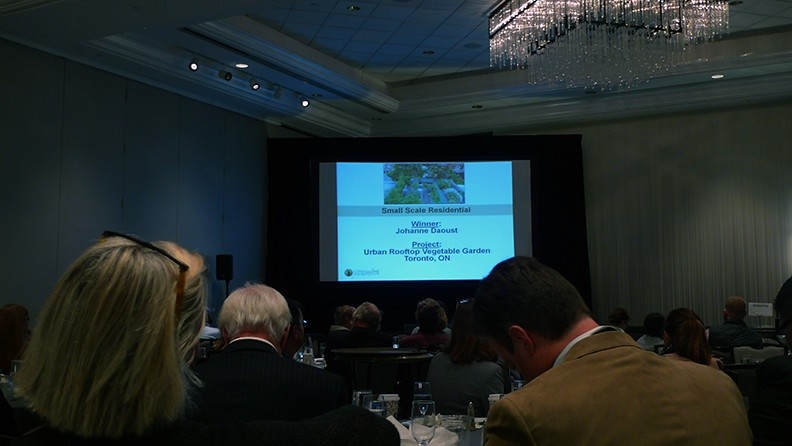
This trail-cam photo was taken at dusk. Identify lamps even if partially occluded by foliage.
[485,1,730,96]
[187,57,310,108]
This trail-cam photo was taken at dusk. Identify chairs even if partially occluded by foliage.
[329,337,792,446]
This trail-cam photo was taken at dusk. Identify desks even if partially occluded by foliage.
[395,413,486,446]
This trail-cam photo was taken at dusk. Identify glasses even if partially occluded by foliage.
[295,320,307,328]
[456,299,471,308]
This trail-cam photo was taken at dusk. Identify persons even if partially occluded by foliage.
[607,307,630,330]
[427,294,510,418]
[471,256,756,446]
[179,282,355,420]
[746,276,792,446]
[0,227,402,446]
[279,298,452,361]
[636,313,666,354]
[0,303,33,375]
[709,295,762,363]
[658,307,725,371]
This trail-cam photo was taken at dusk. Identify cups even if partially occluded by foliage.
[457,417,484,446]
[363,395,386,419]
[488,394,508,408]
[351,390,372,410]
[378,394,400,420]
[293,345,327,370]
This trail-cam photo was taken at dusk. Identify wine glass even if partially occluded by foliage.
[414,381,432,417]
[411,400,436,446]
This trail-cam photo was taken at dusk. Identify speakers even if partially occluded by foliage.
[216,255,234,281]
